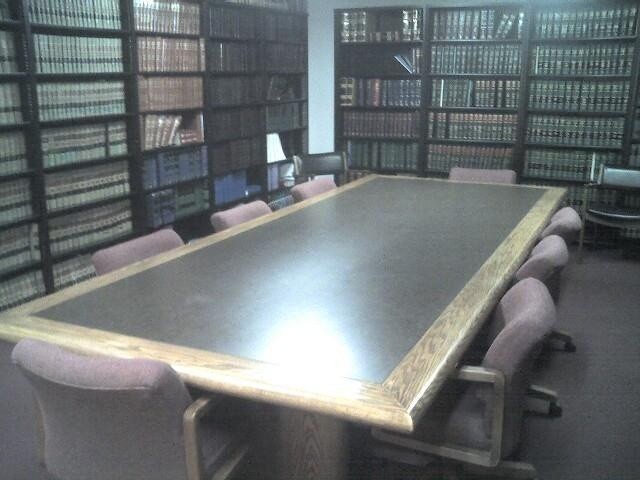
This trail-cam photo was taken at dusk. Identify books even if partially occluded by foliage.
[531,77,638,112]
[426,145,515,172]
[0,121,128,176]
[430,43,523,76]
[208,105,307,141]
[143,149,209,187]
[137,35,206,72]
[141,112,206,146]
[216,155,303,202]
[428,111,517,142]
[565,185,639,240]
[339,8,424,43]
[138,75,205,110]
[206,1,306,43]
[149,182,212,227]
[0,0,125,30]
[0,32,126,76]
[341,110,421,140]
[537,5,640,37]
[527,114,639,145]
[526,148,640,183]
[0,160,132,224]
[1,262,73,311]
[0,198,134,286]
[532,42,633,75]
[214,132,287,170]
[0,79,128,125]
[341,78,422,108]
[343,140,419,170]
[432,78,523,109]
[211,40,304,73]
[129,0,202,34]
[213,74,303,105]
[394,47,422,75]
[432,7,525,39]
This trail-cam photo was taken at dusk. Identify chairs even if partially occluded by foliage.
[363,277,558,480]
[514,235,570,282]
[540,207,581,238]
[292,151,349,192]
[12,338,264,480]
[580,165,640,249]
[92,226,185,274]
[211,199,273,231]
[290,177,339,202]
[446,167,516,187]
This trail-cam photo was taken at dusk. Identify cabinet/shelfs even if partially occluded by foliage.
[0,0,308,310]
[334,1,640,239]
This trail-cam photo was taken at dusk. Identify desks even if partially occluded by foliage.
[0,174,569,480]
